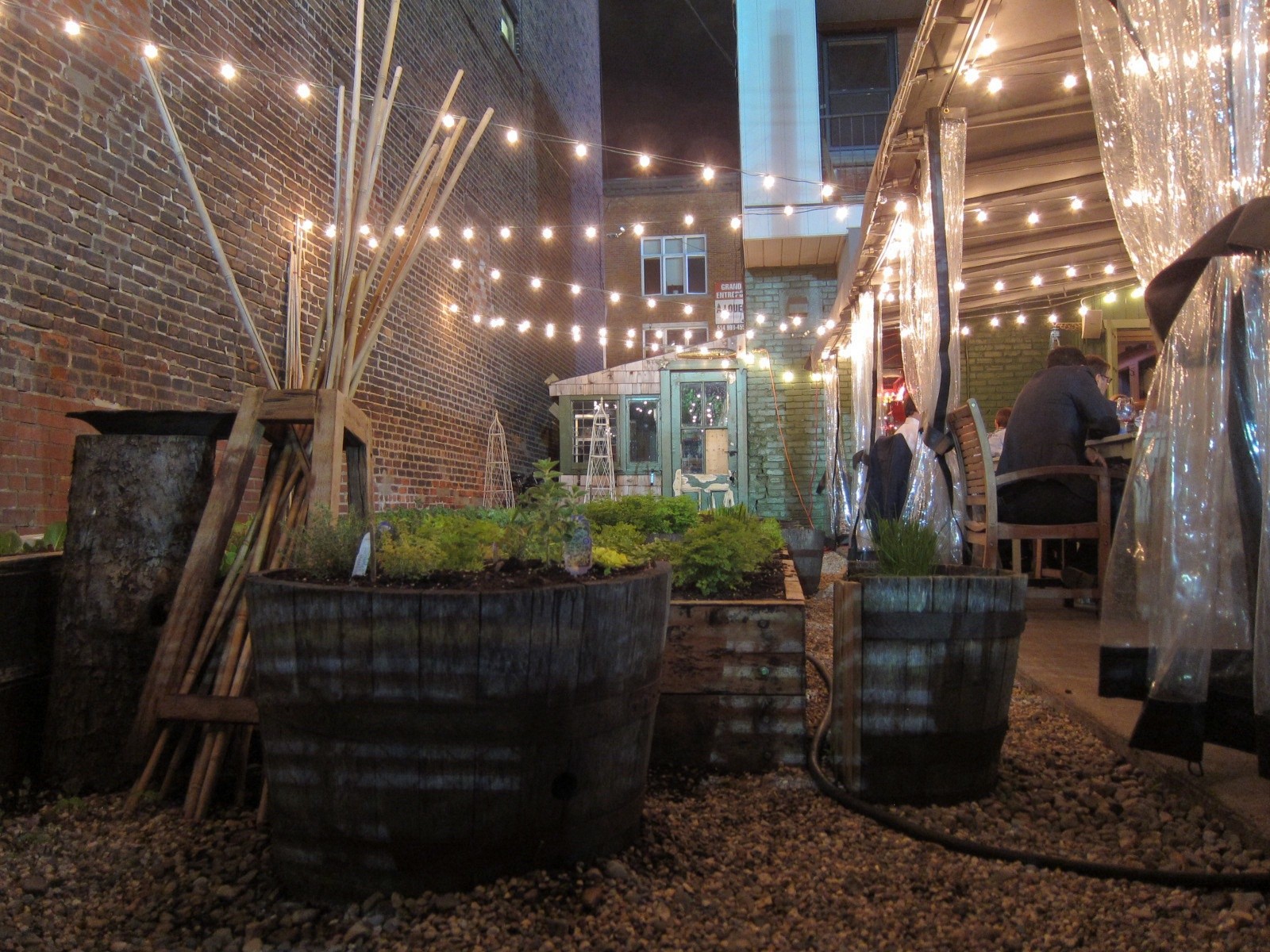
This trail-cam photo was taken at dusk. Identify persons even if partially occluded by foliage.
[989,346,1129,588]
[893,396,919,454]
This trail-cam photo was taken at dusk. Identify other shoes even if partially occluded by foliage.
[1061,567,1096,588]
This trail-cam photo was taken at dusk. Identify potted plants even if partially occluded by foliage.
[240,453,807,902]
[829,502,1028,808]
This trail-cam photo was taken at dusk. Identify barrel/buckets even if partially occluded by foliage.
[782,528,824,597]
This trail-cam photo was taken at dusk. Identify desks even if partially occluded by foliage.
[1084,431,1137,460]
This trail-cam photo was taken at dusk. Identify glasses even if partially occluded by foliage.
[1102,375,1112,385]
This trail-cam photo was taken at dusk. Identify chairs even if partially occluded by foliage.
[945,394,1131,618]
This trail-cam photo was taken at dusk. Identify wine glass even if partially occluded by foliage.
[1117,397,1136,434]
